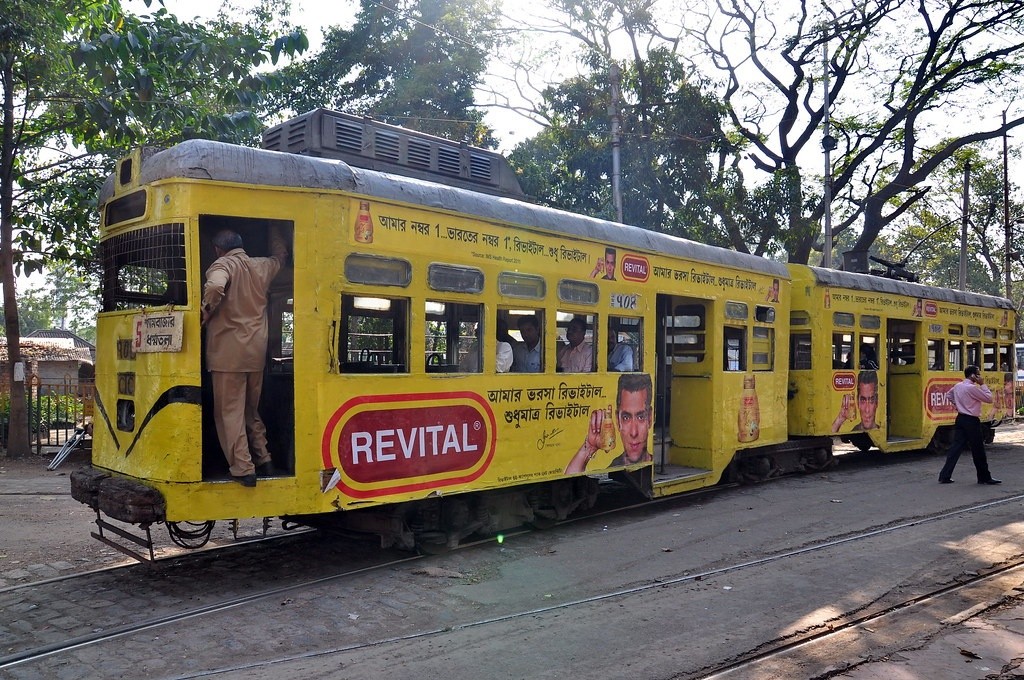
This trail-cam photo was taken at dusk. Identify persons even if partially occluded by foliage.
[556,318,593,373]
[1000,311,1008,327]
[590,248,617,281]
[608,328,634,372]
[912,298,922,317]
[511,315,541,373]
[831,370,879,432]
[988,373,1013,421]
[564,373,653,475]
[496,318,517,349]
[87,418,93,436]
[200,221,288,488]
[462,318,513,373]
[765,279,779,304]
[991,353,1007,371]
[845,343,879,370]
[887,342,915,365]
[938,366,1002,484]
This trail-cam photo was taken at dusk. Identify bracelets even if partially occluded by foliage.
[585,435,596,460]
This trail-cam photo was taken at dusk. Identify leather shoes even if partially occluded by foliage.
[977,477,1002,484]
[939,478,954,483]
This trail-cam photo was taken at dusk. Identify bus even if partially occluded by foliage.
[832,332,1024,381]
[69,109,1015,569]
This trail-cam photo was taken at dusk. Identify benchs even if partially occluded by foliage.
[68,439,93,450]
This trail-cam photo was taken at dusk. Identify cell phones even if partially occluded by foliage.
[971,374,975,378]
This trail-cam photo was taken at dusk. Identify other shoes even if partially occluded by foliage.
[257,462,276,477]
[234,473,256,487]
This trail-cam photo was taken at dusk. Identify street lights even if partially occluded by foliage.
[1003,95,1017,303]
[821,8,863,268]
